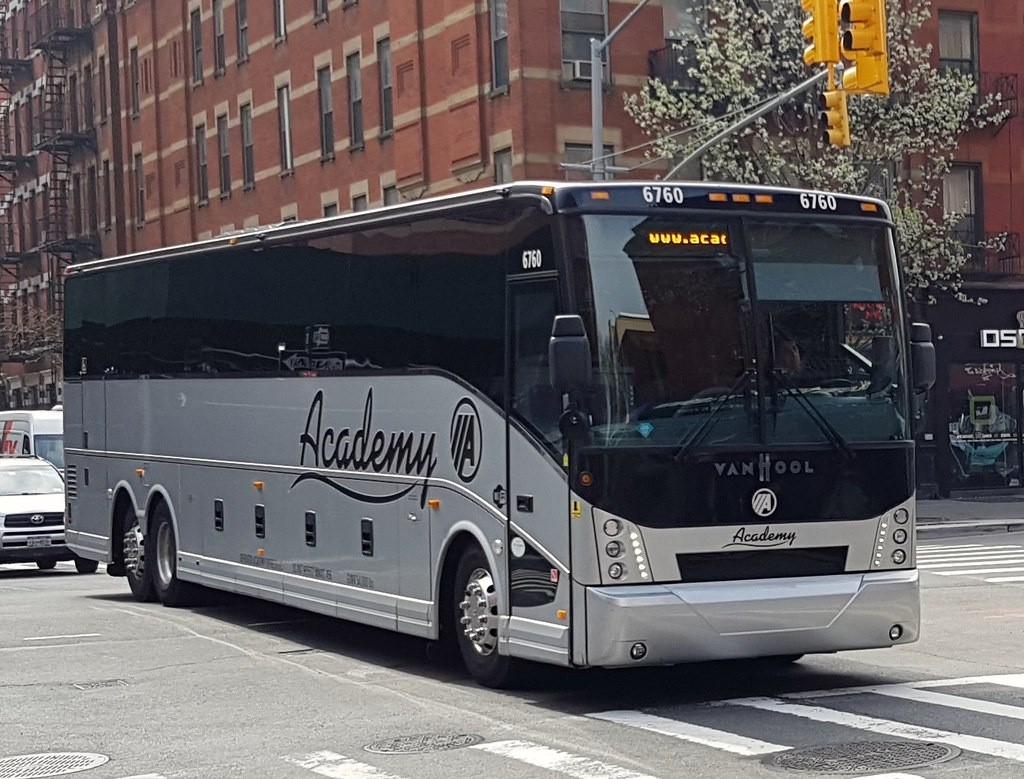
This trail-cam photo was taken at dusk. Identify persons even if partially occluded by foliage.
[37,441,63,465]
[759,331,801,374]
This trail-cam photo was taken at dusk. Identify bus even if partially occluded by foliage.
[62,180,938,689]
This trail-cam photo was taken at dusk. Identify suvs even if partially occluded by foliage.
[0,453,99,575]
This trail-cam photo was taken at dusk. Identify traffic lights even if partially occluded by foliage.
[800,0,840,67]
[819,90,850,150]
[836,0,891,96]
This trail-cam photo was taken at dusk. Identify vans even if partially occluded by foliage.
[0,402,65,481]
